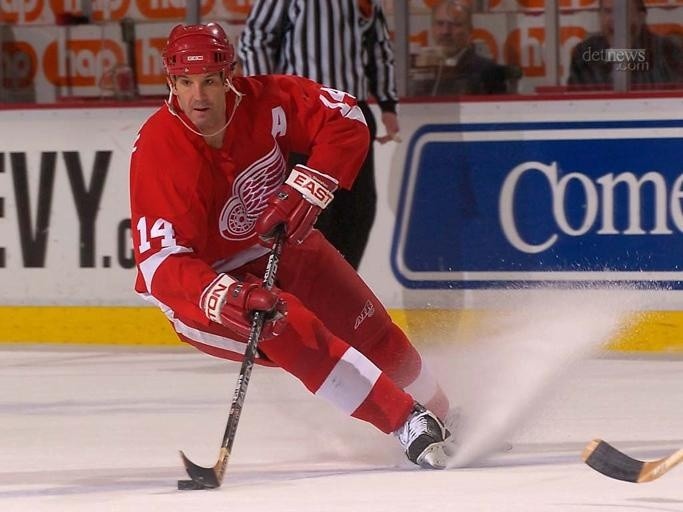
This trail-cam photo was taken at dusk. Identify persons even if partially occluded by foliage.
[407,1,520,99]
[128,22,460,471]
[238,0,401,280]
[563,0,683,87]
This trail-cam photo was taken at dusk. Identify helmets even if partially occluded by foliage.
[162,21,238,90]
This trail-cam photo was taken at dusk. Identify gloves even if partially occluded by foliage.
[198,271,288,341]
[255,163,340,250]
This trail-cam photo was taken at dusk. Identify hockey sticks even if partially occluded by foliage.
[581,439,682,483]
[180,223,285,488]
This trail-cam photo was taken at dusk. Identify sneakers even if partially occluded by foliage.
[396,401,450,466]
